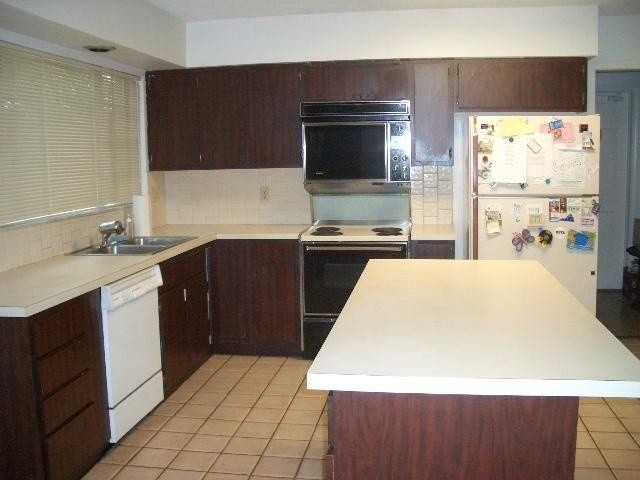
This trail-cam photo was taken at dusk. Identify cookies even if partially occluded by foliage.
[298,100,413,193]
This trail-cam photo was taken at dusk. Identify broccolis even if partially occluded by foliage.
[466,114,602,324]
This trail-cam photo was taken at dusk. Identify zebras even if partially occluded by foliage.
[301,245,409,357]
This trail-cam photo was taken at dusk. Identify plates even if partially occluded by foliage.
[144,54,585,170]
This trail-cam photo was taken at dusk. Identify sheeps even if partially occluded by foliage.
[99,222,124,245]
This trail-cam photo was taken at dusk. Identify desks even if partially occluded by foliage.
[307,256,639,480]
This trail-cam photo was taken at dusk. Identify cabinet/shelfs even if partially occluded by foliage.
[159,241,213,400]
[0,286,111,480]
[208,238,306,357]
[101,264,164,443]
[144,54,585,170]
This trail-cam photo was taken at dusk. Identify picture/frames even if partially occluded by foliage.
[307,256,639,480]
